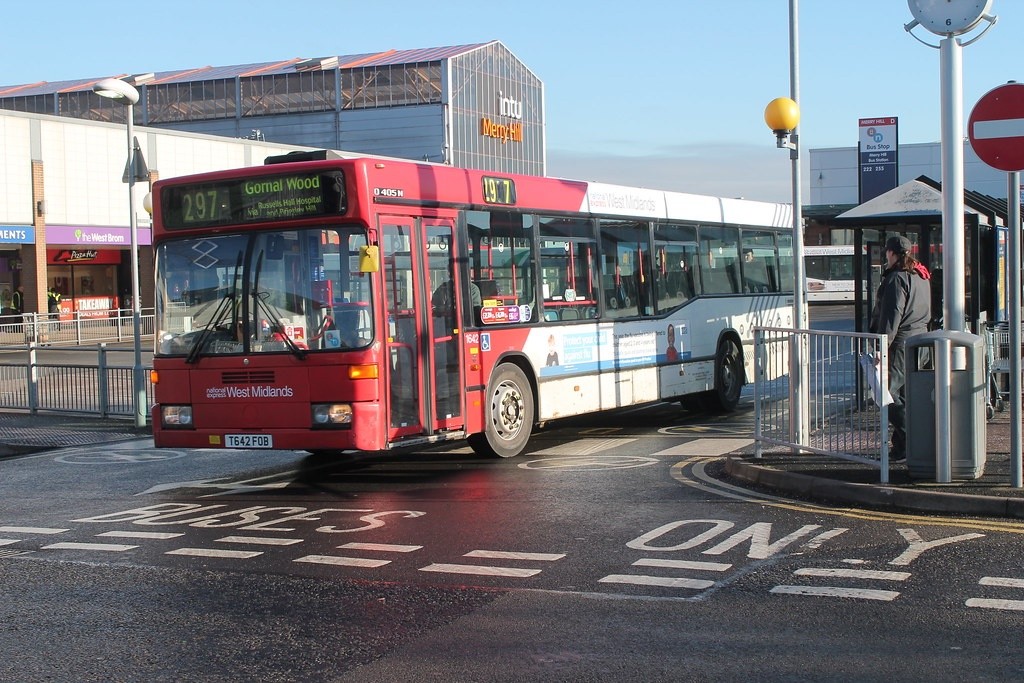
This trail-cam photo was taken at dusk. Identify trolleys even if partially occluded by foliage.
[982,320,1024,419]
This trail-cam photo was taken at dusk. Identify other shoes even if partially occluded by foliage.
[877,454,906,464]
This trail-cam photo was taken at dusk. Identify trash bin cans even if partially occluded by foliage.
[904,330,986,479]
[125,295,142,325]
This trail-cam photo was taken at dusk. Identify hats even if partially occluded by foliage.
[880,237,911,252]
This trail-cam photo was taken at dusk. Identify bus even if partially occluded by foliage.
[148,148,808,463]
[709,248,869,302]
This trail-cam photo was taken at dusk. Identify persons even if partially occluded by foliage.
[667,324,677,362]
[728,247,770,293]
[688,250,729,294]
[545,335,559,367]
[10,286,24,332]
[869,235,931,464]
[48,287,62,331]
[432,257,481,310]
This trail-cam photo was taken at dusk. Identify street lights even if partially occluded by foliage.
[92,79,146,431]
[764,98,808,454]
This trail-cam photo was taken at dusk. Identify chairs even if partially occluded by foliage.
[559,263,778,313]
[470,280,500,326]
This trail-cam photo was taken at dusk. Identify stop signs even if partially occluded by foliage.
[966,83,1024,174]
